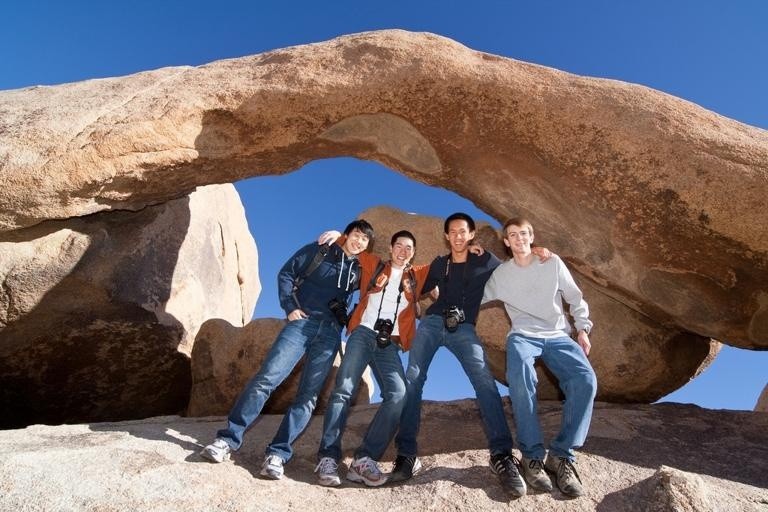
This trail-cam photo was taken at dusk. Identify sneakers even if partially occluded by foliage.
[520,456,553,493]
[261,455,285,480]
[545,454,584,498]
[345,456,387,488]
[201,440,230,463]
[489,454,526,497]
[319,457,343,486]
[389,455,423,481]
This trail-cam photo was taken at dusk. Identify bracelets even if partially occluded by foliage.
[578,326,592,336]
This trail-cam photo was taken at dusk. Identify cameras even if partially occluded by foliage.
[374,319,394,349]
[327,297,349,327]
[442,304,466,333]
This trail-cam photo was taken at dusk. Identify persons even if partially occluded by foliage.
[198,219,375,481]
[427,217,599,497]
[312,230,484,490]
[389,209,553,498]
